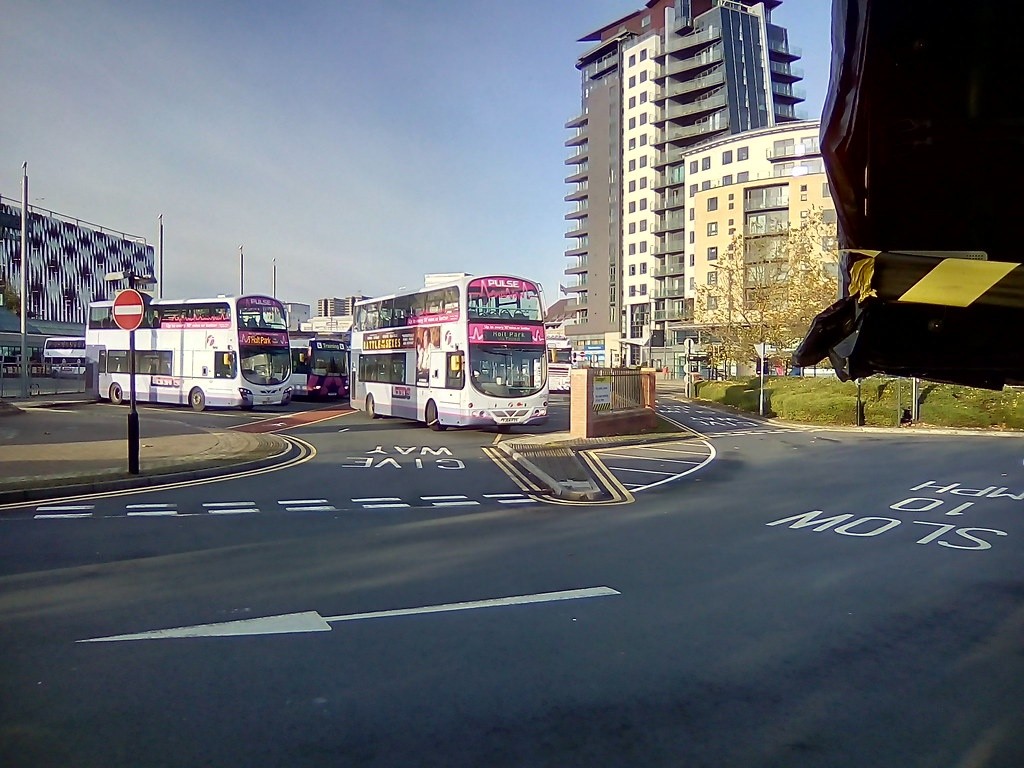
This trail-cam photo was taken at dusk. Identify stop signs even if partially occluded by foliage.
[580,352,585,357]
[112,290,146,334]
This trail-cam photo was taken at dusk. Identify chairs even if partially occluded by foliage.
[259,318,271,328]
[239,318,246,328]
[478,308,525,318]
[152,317,159,327]
[247,318,258,327]
[102,318,150,327]
[362,313,418,330]
[360,364,402,382]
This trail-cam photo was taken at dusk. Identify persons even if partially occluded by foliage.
[662,365,669,381]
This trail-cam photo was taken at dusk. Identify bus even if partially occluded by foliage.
[42,335,86,379]
[82,294,295,413]
[273,337,352,397]
[349,271,558,431]
[546,333,577,392]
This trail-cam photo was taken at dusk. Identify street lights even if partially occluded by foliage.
[708,262,751,380]
